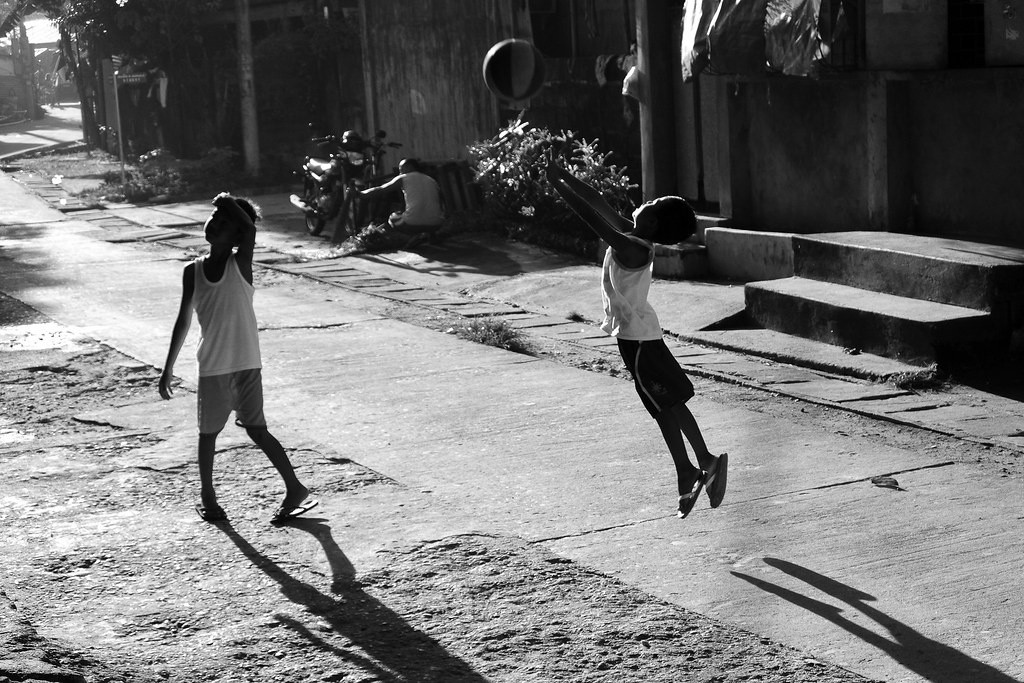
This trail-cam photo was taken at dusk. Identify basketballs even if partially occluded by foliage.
[481,37,550,104]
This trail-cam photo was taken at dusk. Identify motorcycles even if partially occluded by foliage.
[287,122,388,238]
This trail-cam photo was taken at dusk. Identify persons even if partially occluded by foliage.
[358,158,449,238]
[158,192,318,521]
[535,144,728,519]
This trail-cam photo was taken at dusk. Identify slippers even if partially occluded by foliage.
[194,503,228,522]
[269,500,319,523]
[704,453,729,509]
[677,469,708,520]
